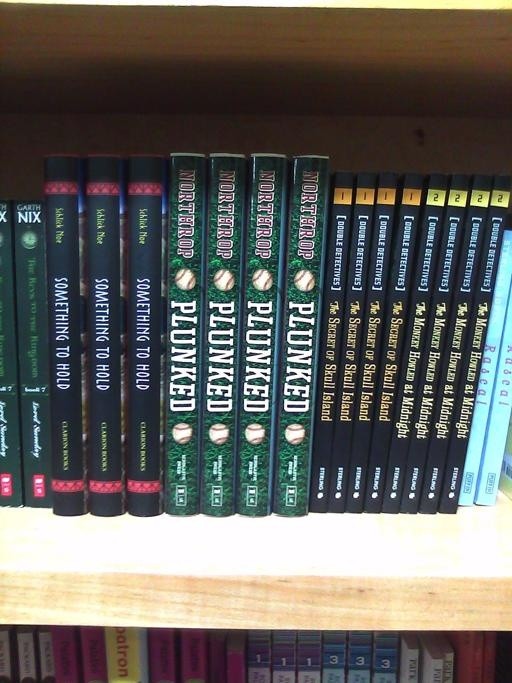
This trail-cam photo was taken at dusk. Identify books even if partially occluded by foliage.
[0,155,512,516]
[0,623,512,683]
